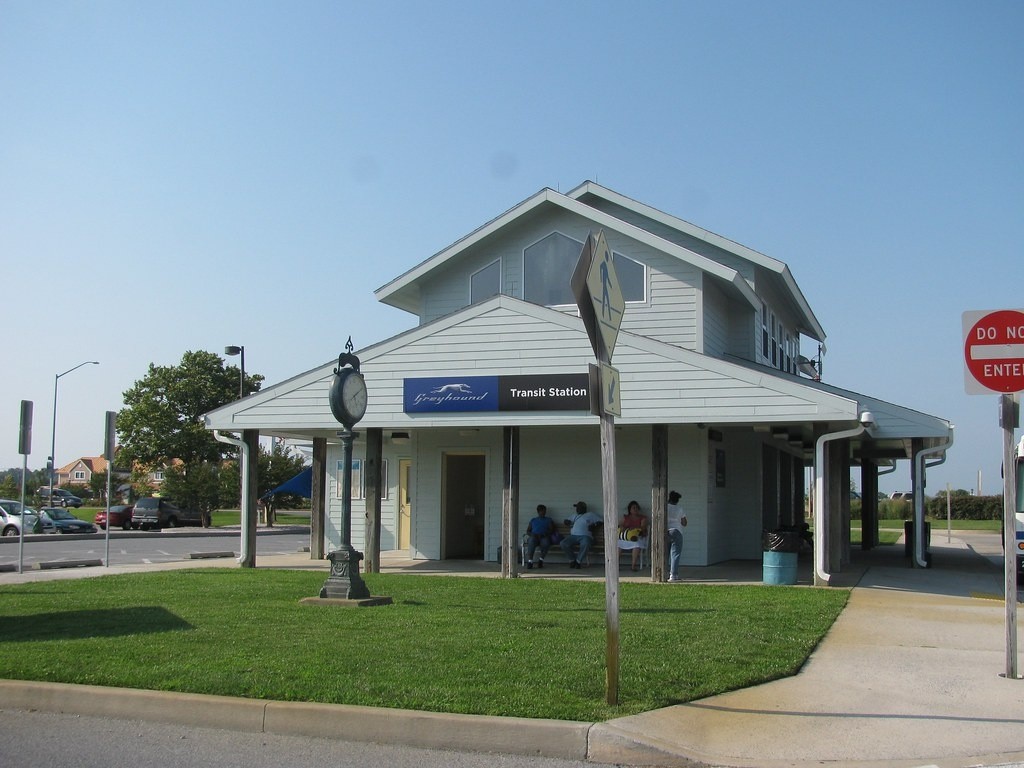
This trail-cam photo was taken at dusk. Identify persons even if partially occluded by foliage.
[560,502,603,570]
[526,505,561,569]
[617,501,648,572]
[666,490,688,583]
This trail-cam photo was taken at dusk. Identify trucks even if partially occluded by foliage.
[1001,434,1024,584]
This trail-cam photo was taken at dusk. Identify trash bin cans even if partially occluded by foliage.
[761,529,801,585]
[904,520,931,568]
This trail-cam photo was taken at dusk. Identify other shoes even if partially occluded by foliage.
[527,562,533,569]
[575,562,581,569]
[570,560,575,567]
[669,575,679,580]
[537,559,543,568]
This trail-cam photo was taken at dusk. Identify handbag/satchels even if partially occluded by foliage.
[551,529,563,544]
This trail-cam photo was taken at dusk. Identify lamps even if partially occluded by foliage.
[786,435,802,447]
[771,428,788,440]
[802,442,814,454]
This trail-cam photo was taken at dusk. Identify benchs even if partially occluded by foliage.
[521,523,650,570]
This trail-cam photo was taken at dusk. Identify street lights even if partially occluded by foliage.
[48,362,100,508]
[225,344,244,400]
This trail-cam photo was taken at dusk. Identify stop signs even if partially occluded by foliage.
[962,310,1024,396]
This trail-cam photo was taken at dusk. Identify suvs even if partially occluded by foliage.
[849,490,913,503]
[34,488,83,509]
[130,497,211,530]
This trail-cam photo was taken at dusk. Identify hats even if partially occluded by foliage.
[669,491,681,498]
[573,502,587,508]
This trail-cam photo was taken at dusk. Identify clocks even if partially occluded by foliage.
[336,371,367,420]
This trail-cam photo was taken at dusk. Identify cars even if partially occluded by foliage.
[95,505,141,530]
[0,499,41,536]
[37,508,98,534]
